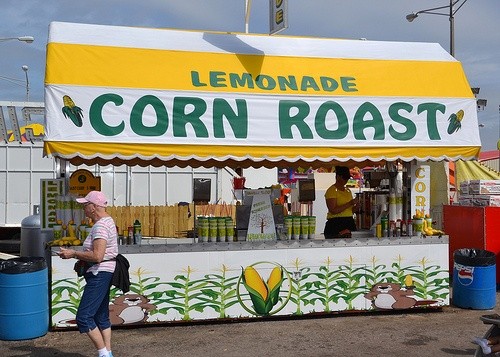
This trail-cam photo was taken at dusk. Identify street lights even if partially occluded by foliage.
[22,65,30,125]
[406,0,467,58]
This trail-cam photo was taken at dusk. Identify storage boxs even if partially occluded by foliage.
[458,180,500,207]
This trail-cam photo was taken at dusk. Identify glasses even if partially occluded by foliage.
[82,201,92,208]
[339,175,350,180]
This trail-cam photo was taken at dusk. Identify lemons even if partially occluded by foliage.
[57,220,95,230]
[53,240,81,246]
[425,227,445,236]
[425,214,430,217]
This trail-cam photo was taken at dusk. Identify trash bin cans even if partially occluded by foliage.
[0,256,49,340]
[452,248,495,310]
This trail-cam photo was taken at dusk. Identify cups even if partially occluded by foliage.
[197,217,233,242]
[120,220,141,246]
[53,224,92,243]
[389,188,403,221]
[283,215,316,240]
[372,217,433,237]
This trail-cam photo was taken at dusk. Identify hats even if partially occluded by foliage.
[75,191,107,208]
[335,166,353,177]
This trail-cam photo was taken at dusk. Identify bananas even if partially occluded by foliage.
[61,237,77,240]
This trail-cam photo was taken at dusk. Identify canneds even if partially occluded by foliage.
[122,226,141,246]
[375,217,401,238]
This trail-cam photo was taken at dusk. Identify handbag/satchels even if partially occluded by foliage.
[73,257,117,277]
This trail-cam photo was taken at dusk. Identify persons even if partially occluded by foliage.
[324,165,361,239]
[59,191,119,357]
[471,335,500,355]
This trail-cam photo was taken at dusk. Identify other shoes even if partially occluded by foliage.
[109,351,113,357]
